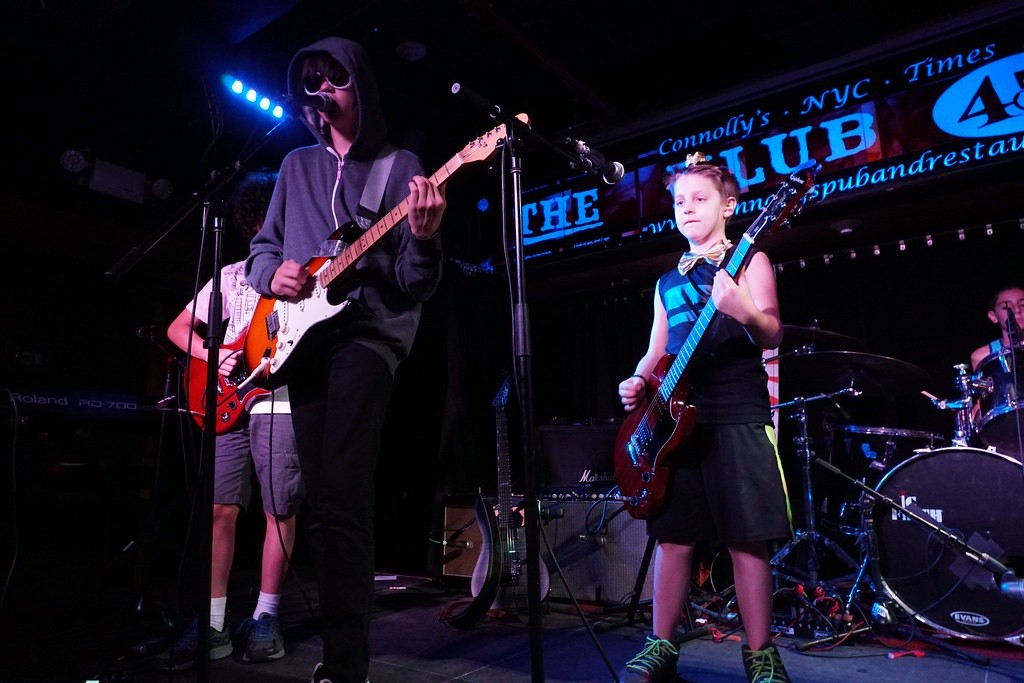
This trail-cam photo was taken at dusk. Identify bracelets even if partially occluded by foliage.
[631,374,647,384]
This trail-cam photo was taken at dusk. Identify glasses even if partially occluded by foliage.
[302,62,352,96]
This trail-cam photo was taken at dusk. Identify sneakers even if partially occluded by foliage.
[237,612,285,660]
[741,642,791,683]
[153,617,234,671]
[311,662,372,683]
[618,632,680,683]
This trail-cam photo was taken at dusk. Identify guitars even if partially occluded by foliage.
[244,112,532,392]
[611,166,814,519]
[471,377,552,610]
[183,321,272,437]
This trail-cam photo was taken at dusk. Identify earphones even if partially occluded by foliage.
[726,205,732,210]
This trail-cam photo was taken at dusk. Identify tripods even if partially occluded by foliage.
[694,365,1007,663]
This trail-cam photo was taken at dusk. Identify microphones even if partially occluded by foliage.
[1006,308,1019,340]
[578,533,607,545]
[443,540,473,549]
[566,137,625,184]
[278,92,332,112]
[1000,568,1024,602]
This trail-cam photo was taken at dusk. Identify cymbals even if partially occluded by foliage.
[782,324,863,345]
[780,351,938,422]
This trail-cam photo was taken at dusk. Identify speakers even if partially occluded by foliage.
[538,492,655,605]
[534,422,625,491]
[443,504,484,578]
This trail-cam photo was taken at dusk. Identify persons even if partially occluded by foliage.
[619,153,793,683]
[970,285,1024,423]
[155,217,307,669]
[244,37,449,683]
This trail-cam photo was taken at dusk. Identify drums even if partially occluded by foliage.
[862,445,1024,641]
[970,341,1024,461]
[819,424,945,537]
